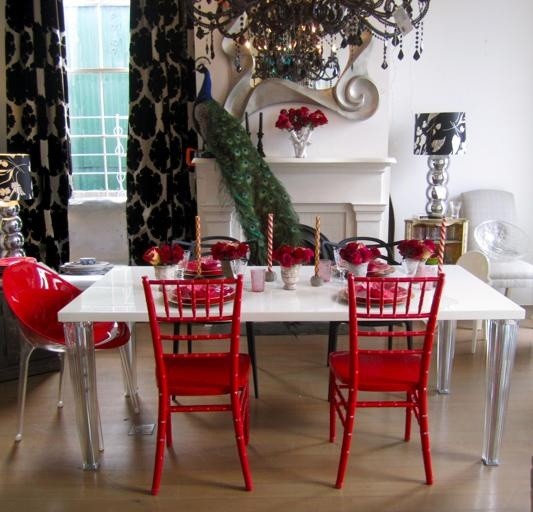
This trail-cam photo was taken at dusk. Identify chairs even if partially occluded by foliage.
[0,260,143,455]
[460,188,533,298]
[436,251,492,353]
[140,275,255,495]
[327,271,447,490]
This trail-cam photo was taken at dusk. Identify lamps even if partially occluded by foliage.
[0,153,35,257]
[413,112,467,216]
[179,1,430,89]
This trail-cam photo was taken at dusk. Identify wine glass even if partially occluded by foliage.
[333,247,346,286]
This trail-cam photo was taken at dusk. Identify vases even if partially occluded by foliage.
[279,264,301,290]
[222,260,249,282]
[404,259,426,277]
[348,262,369,279]
[288,136,312,158]
[154,264,175,291]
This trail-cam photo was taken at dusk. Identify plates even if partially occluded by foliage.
[335,259,414,305]
[167,260,236,305]
[58,260,108,272]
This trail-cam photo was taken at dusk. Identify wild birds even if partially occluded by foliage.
[190,62,303,266]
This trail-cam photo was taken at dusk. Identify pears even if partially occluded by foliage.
[426,257,439,265]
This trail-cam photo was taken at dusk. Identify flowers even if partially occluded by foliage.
[339,241,381,265]
[397,240,436,261]
[143,244,185,266]
[210,242,250,262]
[274,107,328,156]
[271,245,313,267]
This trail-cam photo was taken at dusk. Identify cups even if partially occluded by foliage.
[417,265,439,292]
[400,258,418,277]
[230,259,247,279]
[250,268,266,292]
[178,249,190,270]
[317,258,332,283]
[449,200,462,219]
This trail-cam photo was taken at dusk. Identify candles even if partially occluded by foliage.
[267,213,273,272]
[194,214,201,261]
[437,218,448,274]
[315,215,320,277]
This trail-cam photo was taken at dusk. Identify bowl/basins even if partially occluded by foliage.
[425,259,439,265]
[79,257,95,265]
[474,219,531,259]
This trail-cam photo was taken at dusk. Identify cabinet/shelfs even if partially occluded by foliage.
[0,268,62,382]
[404,217,469,263]
